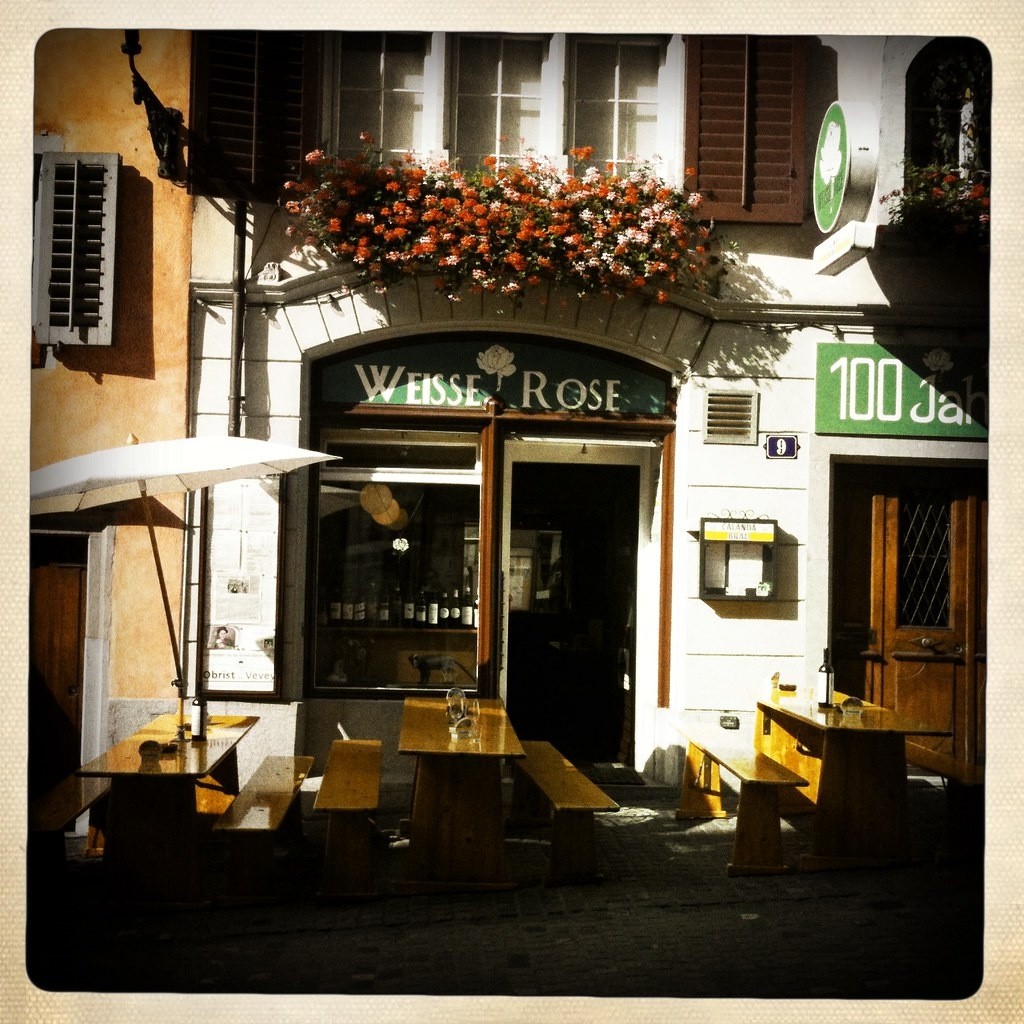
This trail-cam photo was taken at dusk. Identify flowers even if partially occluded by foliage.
[284,133,739,312]
[879,102,991,246]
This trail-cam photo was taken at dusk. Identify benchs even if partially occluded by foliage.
[312,740,384,902]
[27,774,109,912]
[674,727,810,876]
[906,741,984,860]
[210,755,314,908]
[505,740,620,887]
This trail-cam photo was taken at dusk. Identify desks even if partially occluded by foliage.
[72,714,260,912]
[395,696,527,892]
[728,686,952,872]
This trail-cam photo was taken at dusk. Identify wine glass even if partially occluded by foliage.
[446,688,480,743]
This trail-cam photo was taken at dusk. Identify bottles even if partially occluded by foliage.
[426,592,438,628]
[415,591,426,628]
[461,587,473,629]
[403,589,414,628]
[438,592,450,629]
[473,587,479,628]
[317,580,402,627]
[449,589,461,629]
[818,648,834,707]
[191,681,208,742]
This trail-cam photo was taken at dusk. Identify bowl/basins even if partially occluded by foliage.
[160,743,179,753]
[779,684,796,691]
